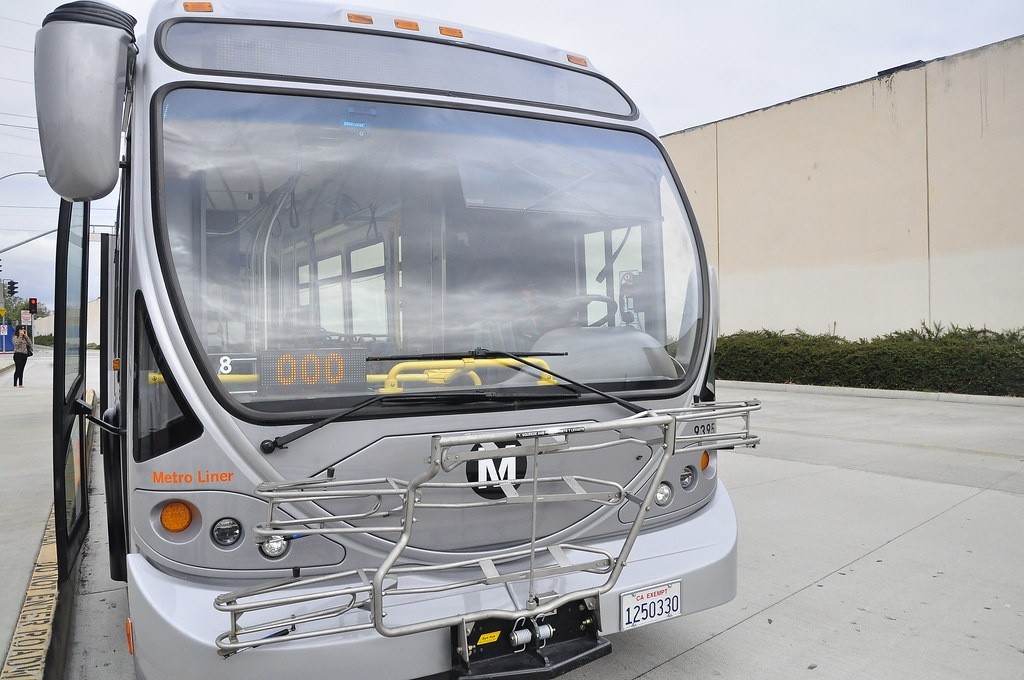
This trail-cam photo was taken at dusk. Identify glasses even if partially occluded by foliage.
[20,328,26,330]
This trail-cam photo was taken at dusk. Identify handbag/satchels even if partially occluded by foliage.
[26,343,33,358]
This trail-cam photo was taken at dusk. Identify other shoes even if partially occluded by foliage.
[14,385,28,389]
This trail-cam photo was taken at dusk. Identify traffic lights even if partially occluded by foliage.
[29,297,37,315]
[0,265,2,267]
[0,270,2,272]
[0,259,1,261]
[12,282,18,296]
[8,280,13,294]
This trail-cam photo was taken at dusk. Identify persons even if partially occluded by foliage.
[12,325,33,388]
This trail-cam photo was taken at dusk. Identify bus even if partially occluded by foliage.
[32,0,762,679]
